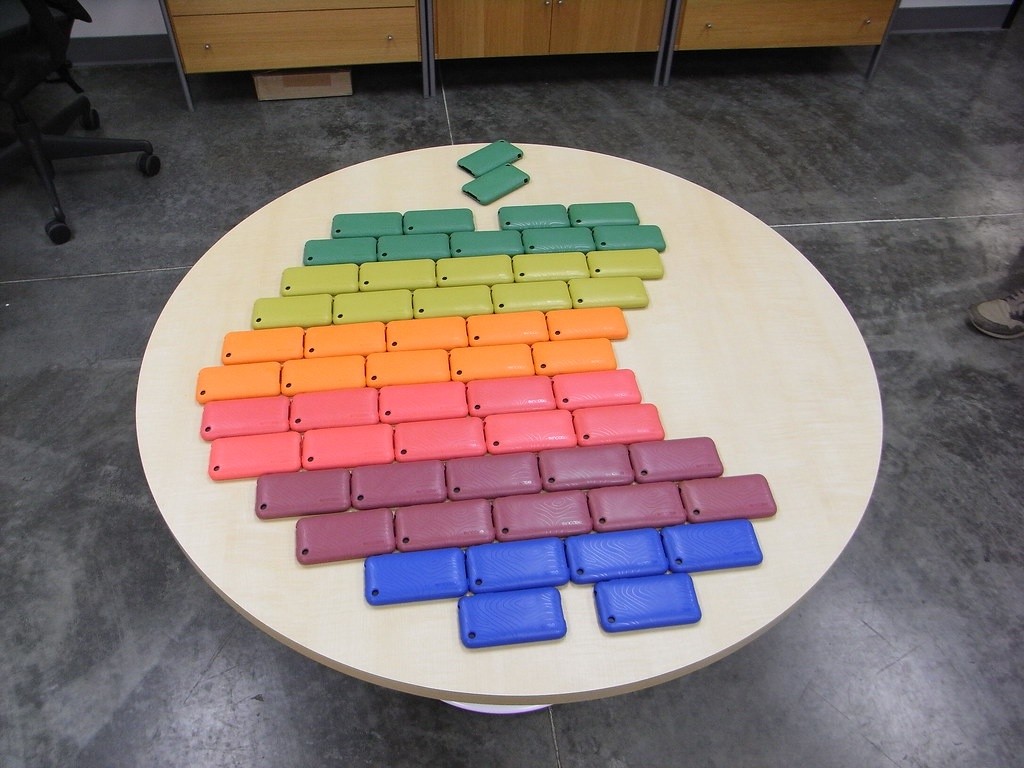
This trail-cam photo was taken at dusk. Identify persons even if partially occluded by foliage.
[969,287,1024,340]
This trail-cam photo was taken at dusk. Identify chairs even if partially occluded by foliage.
[0,0,159,245]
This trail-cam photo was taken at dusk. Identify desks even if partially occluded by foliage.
[135,143,884,713]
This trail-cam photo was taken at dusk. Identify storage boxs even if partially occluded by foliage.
[254,67,354,101]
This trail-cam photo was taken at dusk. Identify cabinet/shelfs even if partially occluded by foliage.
[159,0,901,114]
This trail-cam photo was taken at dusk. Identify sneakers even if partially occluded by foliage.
[967,287,1024,337]
